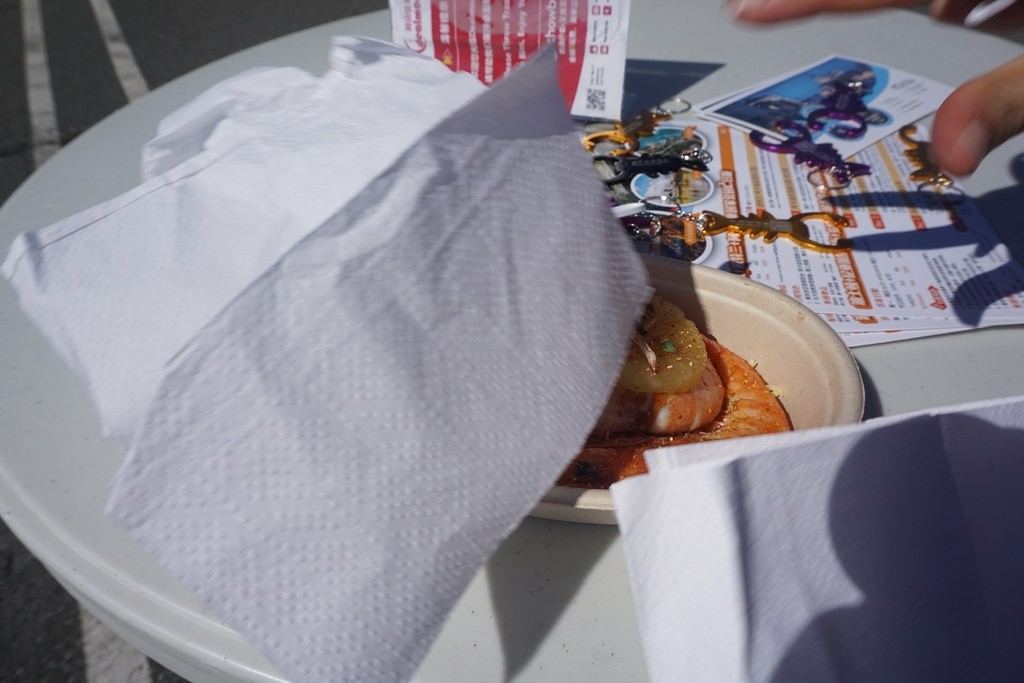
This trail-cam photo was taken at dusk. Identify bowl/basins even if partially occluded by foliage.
[527,250,865,526]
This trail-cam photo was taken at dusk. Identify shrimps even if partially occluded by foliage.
[556,294,801,491]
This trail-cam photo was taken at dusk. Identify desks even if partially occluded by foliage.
[0,1,1024,681]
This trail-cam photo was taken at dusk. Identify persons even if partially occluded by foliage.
[727,0,1024,177]
